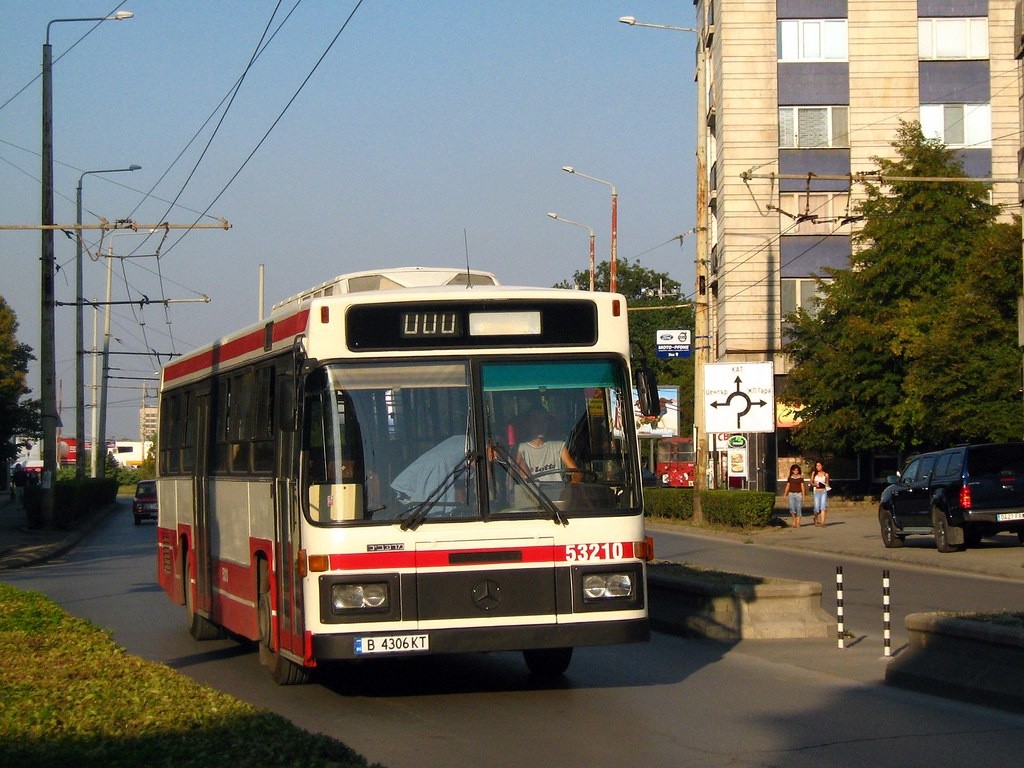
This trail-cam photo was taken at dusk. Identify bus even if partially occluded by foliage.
[656,436,694,489]
[9,459,44,487]
[149,261,664,689]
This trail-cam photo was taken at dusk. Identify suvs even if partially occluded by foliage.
[877,441,1024,553]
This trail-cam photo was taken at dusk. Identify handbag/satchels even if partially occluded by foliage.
[809,471,817,492]
[11,490,16,500]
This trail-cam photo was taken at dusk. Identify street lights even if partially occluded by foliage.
[561,164,619,293]
[74,162,144,521]
[547,211,595,293]
[37,9,138,537]
[94,228,161,475]
[618,15,716,364]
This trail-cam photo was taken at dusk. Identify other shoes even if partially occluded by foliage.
[796,521,800,528]
[792,519,796,528]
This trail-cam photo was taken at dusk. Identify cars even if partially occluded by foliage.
[132,478,158,526]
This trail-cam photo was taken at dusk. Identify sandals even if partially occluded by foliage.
[821,523,826,528]
[814,518,818,528]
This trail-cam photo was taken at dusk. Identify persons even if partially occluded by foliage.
[783,464,806,527]
[810,460,829,527]
[516,404,581,483]
[390,435,474,516]
[29,468,39,486]
[13,464,27,507]
